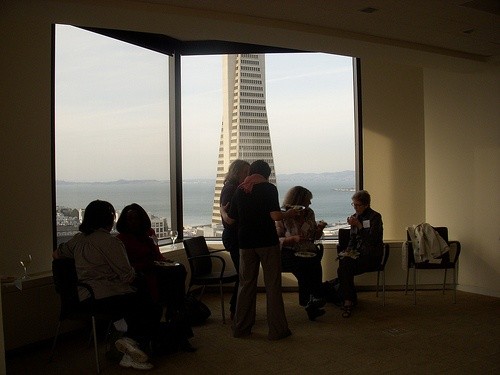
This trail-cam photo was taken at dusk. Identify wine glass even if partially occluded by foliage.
[170,231,178,248]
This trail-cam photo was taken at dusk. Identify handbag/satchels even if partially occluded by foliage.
[322,277,344,305]
[183,293,211,327]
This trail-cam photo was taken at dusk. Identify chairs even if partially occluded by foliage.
[336,228,390,308]
[43,250,119,375]
[182,235,238,324]
[278,238,326,305]
[401,226,461,306]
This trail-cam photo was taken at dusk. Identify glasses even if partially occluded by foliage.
[351,201,363,207]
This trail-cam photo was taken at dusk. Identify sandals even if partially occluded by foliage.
[341,302,353,318]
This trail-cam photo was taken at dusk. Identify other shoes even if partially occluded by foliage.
[120,354,154,370]
[305,305,325,321]
[114,337,148,362]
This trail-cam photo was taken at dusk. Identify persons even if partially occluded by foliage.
[222,160,293,339]
[276,186,323,306]
[337,190,383,317]
[115,204,185,321]
[220,160,251,319]
[53,200,163,370]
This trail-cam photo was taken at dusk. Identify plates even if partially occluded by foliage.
[295,252,316,257]
[281,205,306,211]
